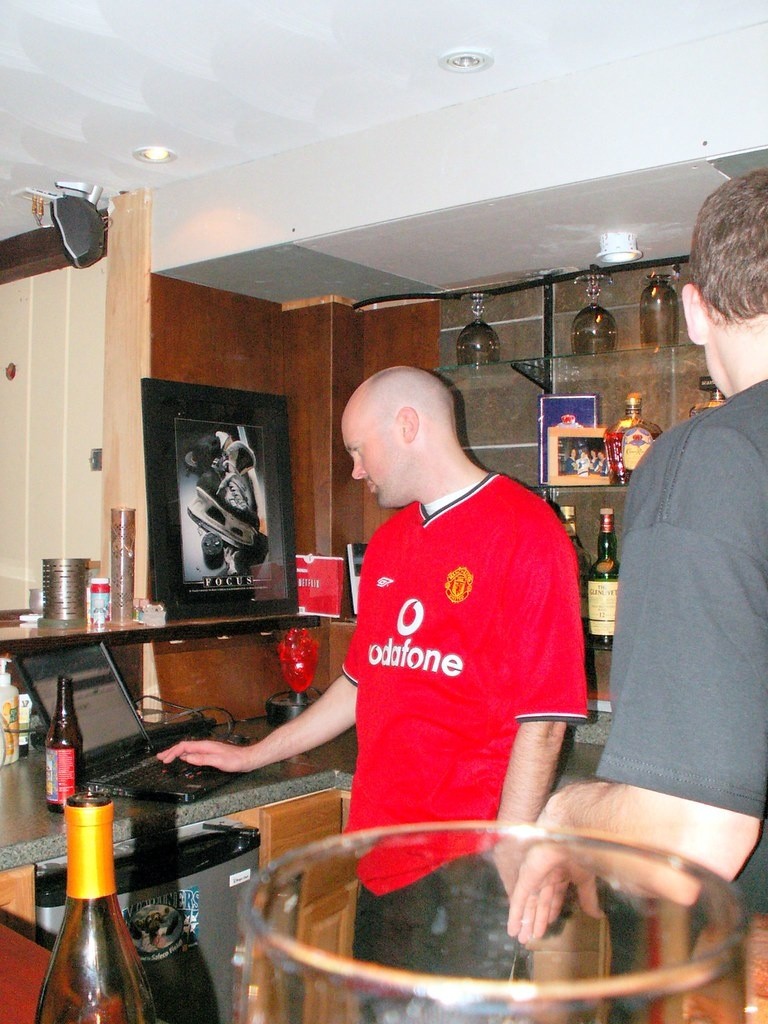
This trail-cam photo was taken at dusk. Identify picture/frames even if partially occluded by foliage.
[141,378,300,621]
[547,427,611,486]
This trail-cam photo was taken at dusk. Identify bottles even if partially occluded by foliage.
[45,676,83,811]
[87,578,110,624]
[602,398,660,484]
[557,506,590,624]
[1,658,20,765]
[640,273,679,347]
[15,682,30,756]
[33,792,158,1024]
[587,507,620,647]
[690,387,728,417]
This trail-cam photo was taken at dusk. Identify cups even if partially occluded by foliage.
[232,820,748,1024]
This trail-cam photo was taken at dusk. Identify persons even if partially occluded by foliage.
[153,366,593,1024]
[506,167,768,947]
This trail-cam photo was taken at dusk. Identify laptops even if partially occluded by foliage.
[11,639,238,802]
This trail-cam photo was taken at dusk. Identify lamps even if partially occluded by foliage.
[49,180,113,270]
[596,231,643,264]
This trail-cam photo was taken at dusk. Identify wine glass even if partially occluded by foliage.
[571,274,617,354]
[456,293,500,366]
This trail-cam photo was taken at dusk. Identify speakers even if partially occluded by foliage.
[49,196,105,269]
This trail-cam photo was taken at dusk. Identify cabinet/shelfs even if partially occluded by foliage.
[0,863,36,943]
[432,341,713,650]
[223,789,359,1024]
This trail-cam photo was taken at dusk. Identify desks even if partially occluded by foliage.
[0,907,129,1024]
